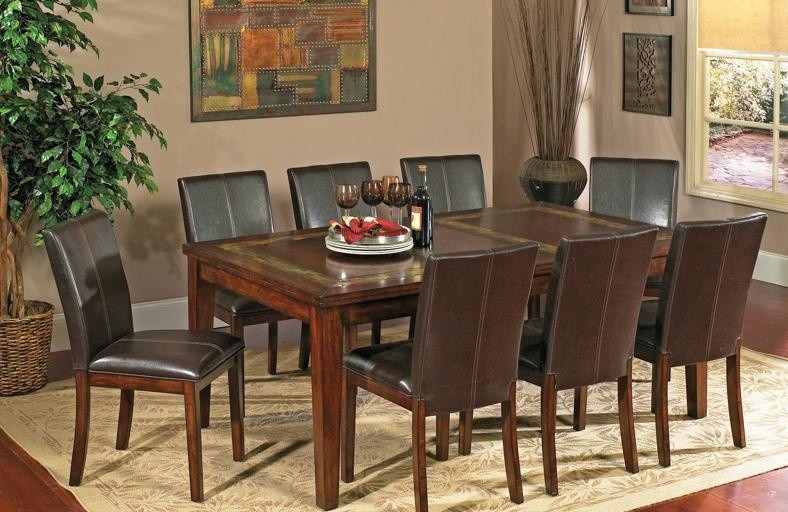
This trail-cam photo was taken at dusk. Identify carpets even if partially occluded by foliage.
[2,322,788,511]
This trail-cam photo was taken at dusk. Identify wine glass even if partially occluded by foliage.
[386,182,410,225]
[334,184,359,215]
[382,176,399,222]
[361,181,383,217]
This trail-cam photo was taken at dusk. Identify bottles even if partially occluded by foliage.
[410,164,433,247]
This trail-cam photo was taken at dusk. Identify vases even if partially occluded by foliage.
[518,156,587,209]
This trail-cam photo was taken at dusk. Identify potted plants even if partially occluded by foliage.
[0,1,167,402]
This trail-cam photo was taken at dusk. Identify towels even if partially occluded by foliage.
[328,214,408,243]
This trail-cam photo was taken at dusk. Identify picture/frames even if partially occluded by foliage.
[186,0,377,122]
[620,31,673,118]
[623,0,672,16]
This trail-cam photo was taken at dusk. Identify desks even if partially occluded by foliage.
[177,195,712,509]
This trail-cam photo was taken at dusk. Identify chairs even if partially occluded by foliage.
[337,242,538,512]
[176,169,297,374]
[39,209,249,503]
[286,161,382,365]
[459,226,657,497]
[397,153,488,217]
[574,211,769,470]
[589,156,682,231]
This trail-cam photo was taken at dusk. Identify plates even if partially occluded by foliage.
[322,236,414,256]
